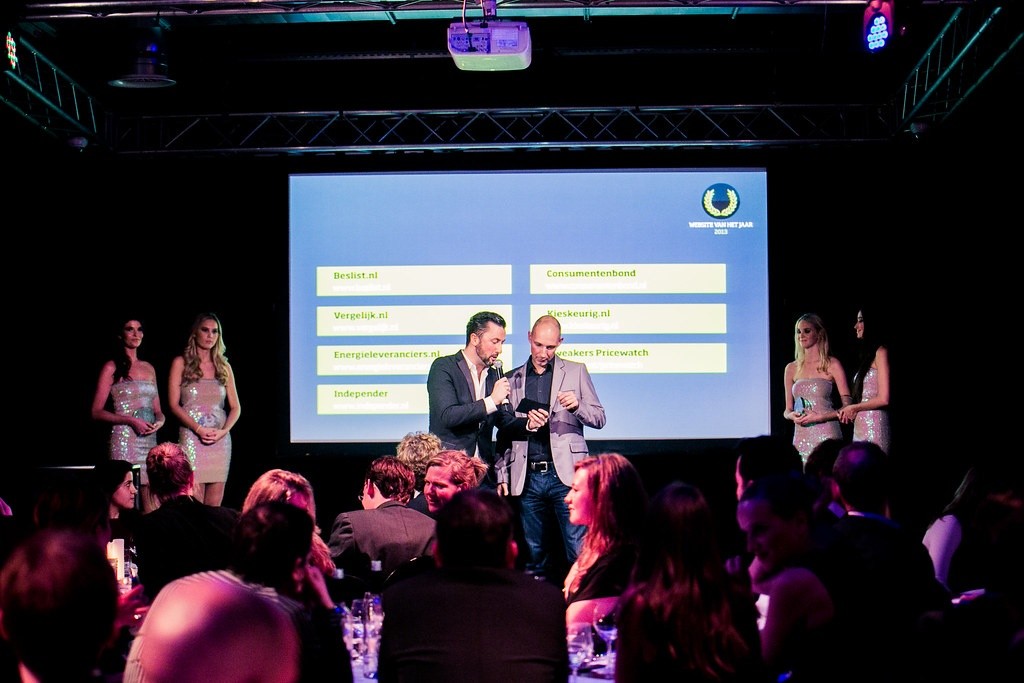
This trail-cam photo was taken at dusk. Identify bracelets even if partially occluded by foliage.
[196,424,201,433]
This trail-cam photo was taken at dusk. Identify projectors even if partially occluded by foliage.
[447,20,531,71]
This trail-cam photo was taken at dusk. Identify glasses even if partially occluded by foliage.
[358,483,367,501]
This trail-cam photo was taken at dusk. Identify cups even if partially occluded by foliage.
[336,593,385,679]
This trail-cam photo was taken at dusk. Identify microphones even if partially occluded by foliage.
[493,360,509,405]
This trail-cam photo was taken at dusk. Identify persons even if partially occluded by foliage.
[0,434,1024,683]
[167,312,243,507]
[426,310,551,489]
[90,313,166,487]
[783,307,891,475]
[493,314,607,578]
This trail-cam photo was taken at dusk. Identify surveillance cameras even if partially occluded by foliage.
[910,123,928,141]
[68,137,87,153]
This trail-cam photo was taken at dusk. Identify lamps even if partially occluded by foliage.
[107,11,176,89]
[863,0,894,53]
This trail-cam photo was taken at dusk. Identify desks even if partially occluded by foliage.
[40,464,140,510]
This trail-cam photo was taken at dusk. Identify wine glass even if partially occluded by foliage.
[565,601,618,682]
